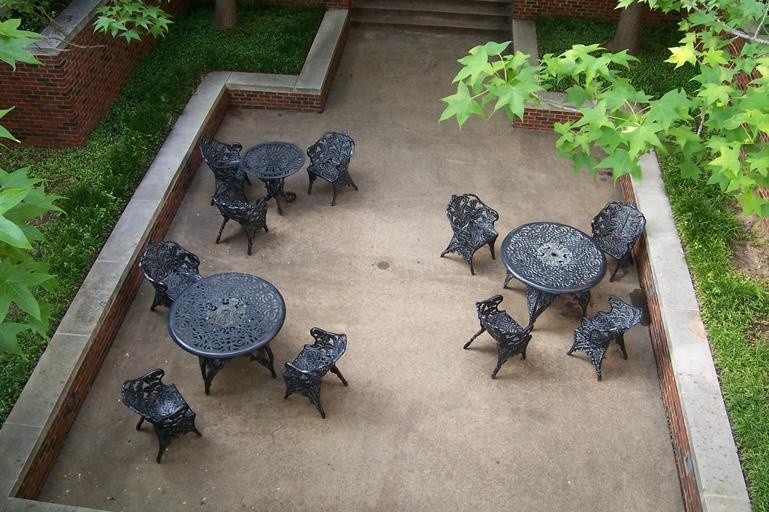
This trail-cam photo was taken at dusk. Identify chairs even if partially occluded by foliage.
[300,129,358,207]
[211,186,268,255]
[283,327,347,417]
[138,239,200,311]
[199,138,252,205]
[464,294,534,380]
[441,193,499,277]
[566,295,643,383]
[591,202,646,283]
[121,368,202,463]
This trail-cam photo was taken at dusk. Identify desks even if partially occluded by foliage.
[167,272,286,396]
[501,222,607,329]
[241,142,305,217]
